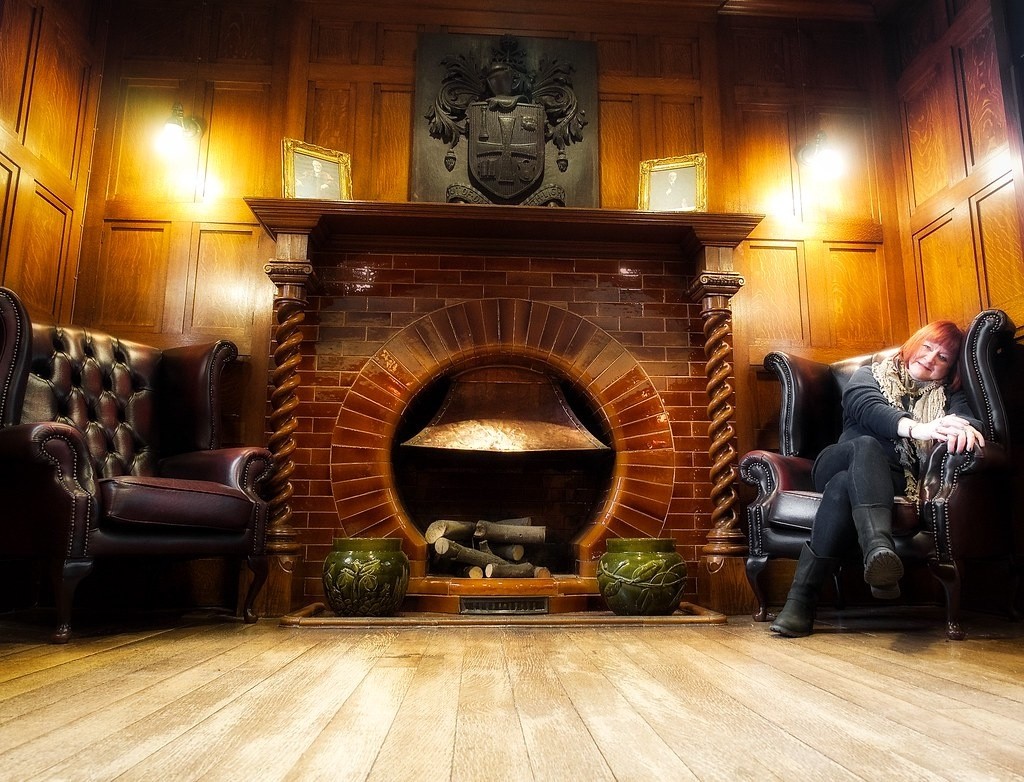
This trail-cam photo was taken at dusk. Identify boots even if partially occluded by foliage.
[770,540,837,638]
[854,503,905,601]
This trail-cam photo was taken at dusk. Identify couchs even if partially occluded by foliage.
[0,285,275,644]
[736,310,1023,640]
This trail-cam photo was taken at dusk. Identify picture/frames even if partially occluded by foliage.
[637,151,706,213]
[279,135,354,201]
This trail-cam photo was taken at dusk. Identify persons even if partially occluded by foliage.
[297,159,337,199]
[655,171,691,212]
[767,317,986,639]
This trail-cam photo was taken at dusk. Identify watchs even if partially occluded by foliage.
[908,420,920,441]
